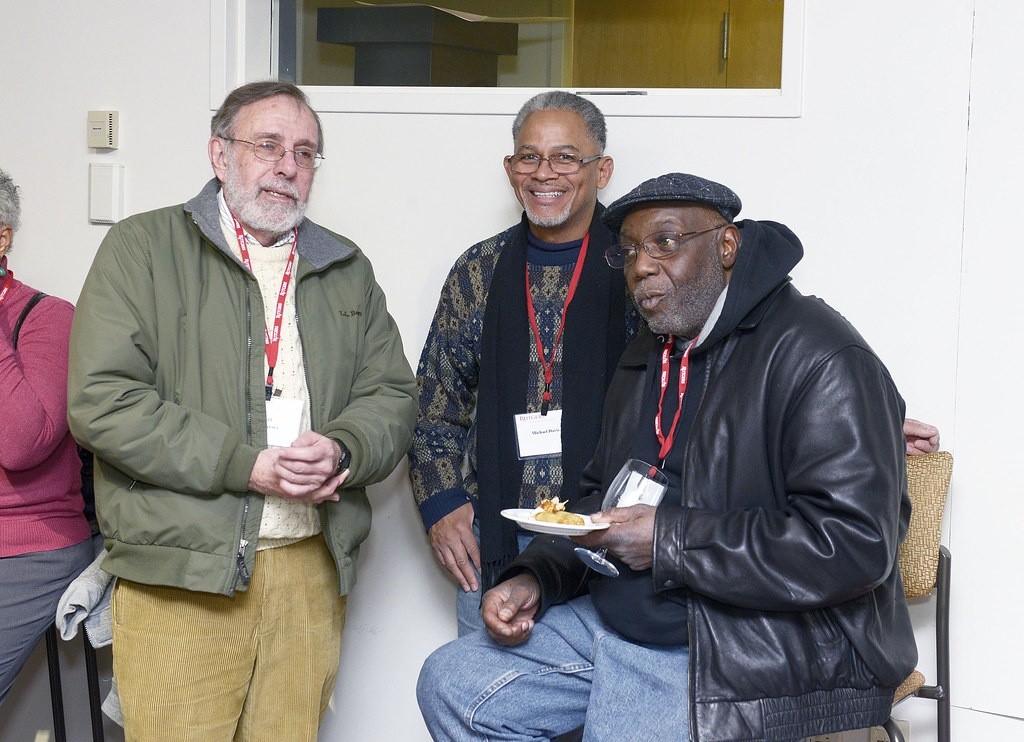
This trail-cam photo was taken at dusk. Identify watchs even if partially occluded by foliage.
[330,437,351,476]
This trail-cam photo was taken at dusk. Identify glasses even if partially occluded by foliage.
[511,152,601,174]
[603,224,727,259]
[222,135,326,170]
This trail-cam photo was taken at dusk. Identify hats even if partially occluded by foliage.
[601,173,743,222]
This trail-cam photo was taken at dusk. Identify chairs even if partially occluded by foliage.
[894,450,951,742]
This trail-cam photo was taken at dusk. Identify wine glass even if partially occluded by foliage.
[574,459,669,576]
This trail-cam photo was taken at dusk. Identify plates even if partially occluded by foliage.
[500,509,611,535]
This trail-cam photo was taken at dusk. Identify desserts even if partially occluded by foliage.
[531,496,585,526]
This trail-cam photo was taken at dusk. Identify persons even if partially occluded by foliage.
[66,83,416,742]
[404,92,940,637]
[0,167,90,703]
[417,173,919,742]
